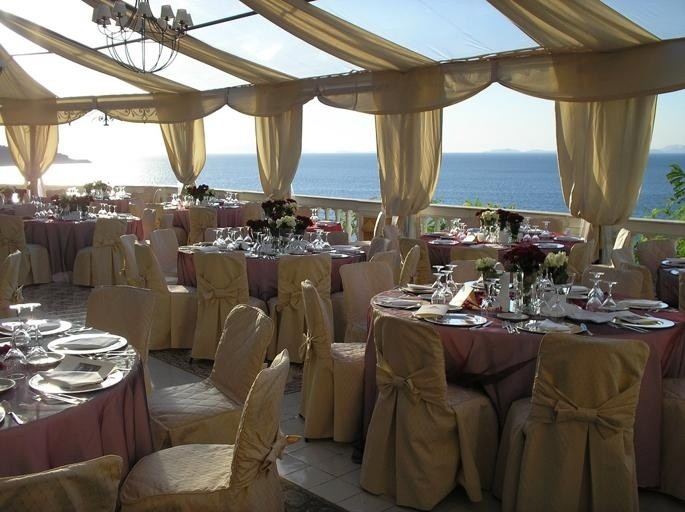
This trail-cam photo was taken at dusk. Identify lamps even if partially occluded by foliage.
[92,0,194,74]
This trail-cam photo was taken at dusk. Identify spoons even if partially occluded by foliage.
[581,322,594,336]
[31,393,80,404]
[3,400,23,425]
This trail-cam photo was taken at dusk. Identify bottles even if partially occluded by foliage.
[514,256,525,289]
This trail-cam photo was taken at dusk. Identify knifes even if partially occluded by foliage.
[481,321,493,328]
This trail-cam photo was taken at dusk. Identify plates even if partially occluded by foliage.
[424,313,487,326]
[375,299,420,308]
[624,316,675,328]
[29,368,123,393]
[569,284,590,300]
[554,235,584,242]
[0,407,5,422]
[618,298,669,309]
[534,242,564,249]
[0,378,16,392]
[470,280,514,291]
[27,352,65,366]
[516,321,583,333]
[47,334,127,355]
[0,319,72,335]
[497,312,528,320]
[429,238,459,245]
[568,311,597,319]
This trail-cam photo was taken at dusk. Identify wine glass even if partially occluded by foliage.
[430,264,458,304]
[33,201,118,218]
[533,266,571,320]
[212,226,334,255]
[64,186,127,199]
[585,270,621,310]
[170,192,238,207]
[3,302,48,381]
[309,208,319,224]
[448,217,551,242]
[1,187,30,204]
